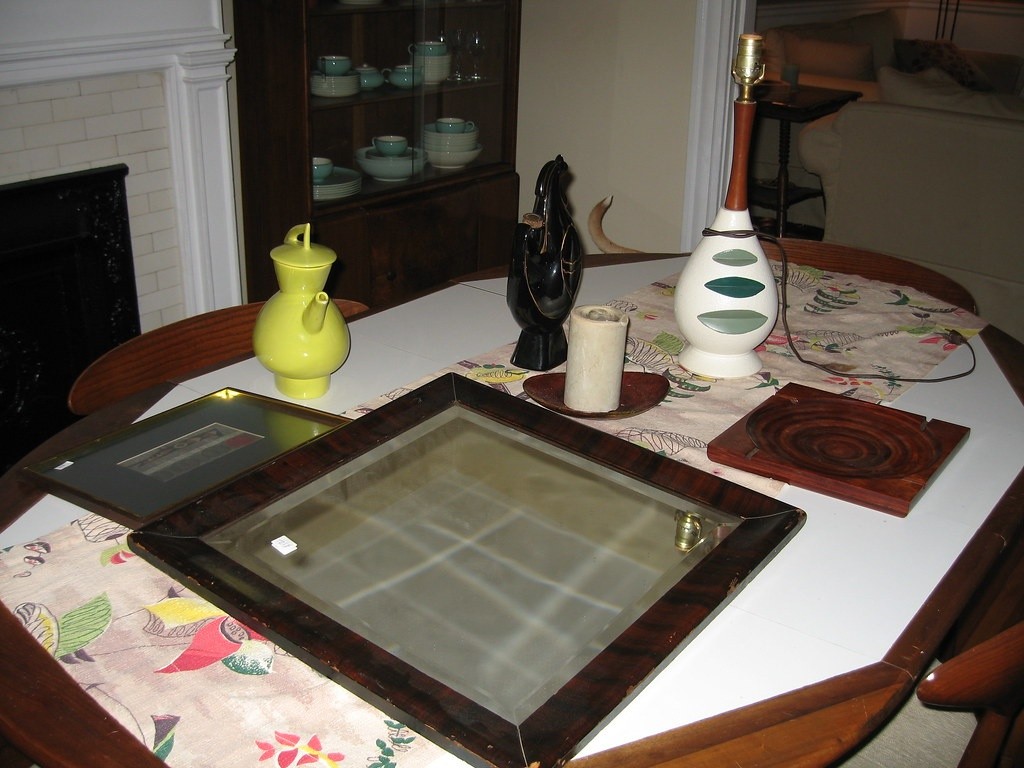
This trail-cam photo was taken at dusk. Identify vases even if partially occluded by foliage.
[674,207,780,379]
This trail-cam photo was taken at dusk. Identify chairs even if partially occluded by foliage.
[826,622,1024,768]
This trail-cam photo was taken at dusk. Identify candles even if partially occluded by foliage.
[563,305,629,414]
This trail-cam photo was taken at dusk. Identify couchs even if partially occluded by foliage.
[752,8,1024,344]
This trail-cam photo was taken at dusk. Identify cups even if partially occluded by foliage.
[781,63,801,86]
[436,117,474,133]
[372,136,408,157]
[564,305,629,412]
[316,55,351,76]
[409,41,448,55]
[313,157,333,182]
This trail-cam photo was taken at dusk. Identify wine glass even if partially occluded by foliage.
[435,29,489,80]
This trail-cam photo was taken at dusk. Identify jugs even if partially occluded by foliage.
[252,224,350,398]
[381,65,425,89]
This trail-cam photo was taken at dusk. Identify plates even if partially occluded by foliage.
[310,71,361,97]
[313,167,361,201]
[420,122,479,151]
[365,148,417,160]
[412,51,452,84]
[523,371,669,420]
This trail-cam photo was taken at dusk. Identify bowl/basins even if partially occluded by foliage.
[356,146,427,182]
[415,142,483,169]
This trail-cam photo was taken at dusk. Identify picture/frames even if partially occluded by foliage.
[17,386,353,530]
[126,372,807,768]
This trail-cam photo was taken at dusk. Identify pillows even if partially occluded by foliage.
[893,36,990,91]
[875,65,1024,120]
[783,33,874,83]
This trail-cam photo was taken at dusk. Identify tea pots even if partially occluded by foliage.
[356,64,385,91]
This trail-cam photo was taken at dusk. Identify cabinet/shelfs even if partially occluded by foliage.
[232,0,522,308]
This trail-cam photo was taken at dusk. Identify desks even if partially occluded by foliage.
[0,255,1024,768]
[748,79,863,241]
